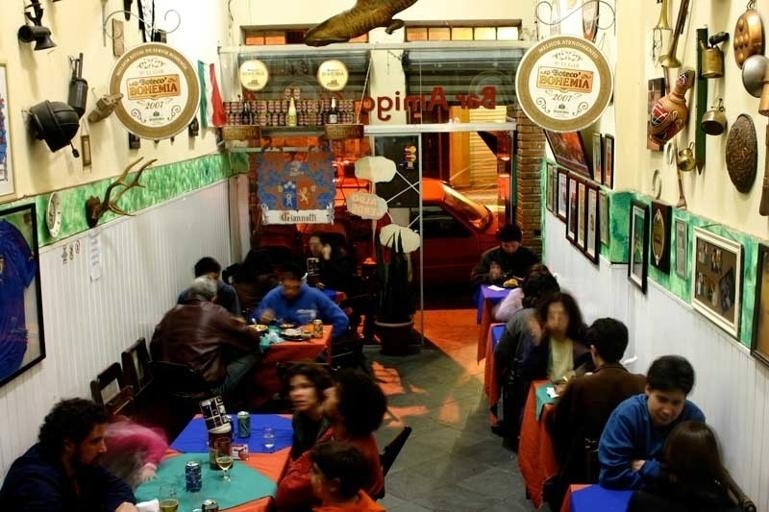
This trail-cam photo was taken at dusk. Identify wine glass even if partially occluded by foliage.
[214,436,235,481]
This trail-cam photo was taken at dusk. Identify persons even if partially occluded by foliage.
[246,270,348,344]
[193,256,240,317]
[308,445,387,512]
[95,419,169,494]
[149,275,263,412]
[505,293,735,512]
[470,226,538,284]
[495,264,560,338]
[274,377,385,510]
[304,232,347,287]
[1,399,138,512]
[278,361,334,459]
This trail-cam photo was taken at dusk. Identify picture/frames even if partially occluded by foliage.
[0,203,48,388]
[750,242,769,369]
[0,62,13,196]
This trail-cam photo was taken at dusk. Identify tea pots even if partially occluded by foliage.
[699,39,723,78]
[700,97,728,136]
[676,141,695,172]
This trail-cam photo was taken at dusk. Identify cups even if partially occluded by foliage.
[261,424,276,449]
[159,483,179,511]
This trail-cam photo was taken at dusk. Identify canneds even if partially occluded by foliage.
[200,500,219,512]
[312,319,324,338]
[184,461,203,492]
[237,411,251,438]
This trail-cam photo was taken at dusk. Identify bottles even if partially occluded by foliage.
[78,117,94,171]
[287,97,297,126]
[328,98,338,125]
[242,102,250,124]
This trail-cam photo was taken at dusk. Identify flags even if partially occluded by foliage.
[197,60,228,129]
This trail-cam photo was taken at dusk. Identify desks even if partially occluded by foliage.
[477,276,754,512]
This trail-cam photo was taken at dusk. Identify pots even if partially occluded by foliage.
[732,0,764,69]
[741,54,768,97]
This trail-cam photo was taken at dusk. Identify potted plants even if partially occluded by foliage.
[373,232,414,354]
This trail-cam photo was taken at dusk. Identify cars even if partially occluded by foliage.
[249,174,505,290]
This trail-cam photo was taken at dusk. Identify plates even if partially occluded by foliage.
[43,192,64,239]
[280,332,305,341]
[250,324,268,332]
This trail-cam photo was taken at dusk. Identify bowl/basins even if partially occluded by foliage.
[279,322,297,329]
